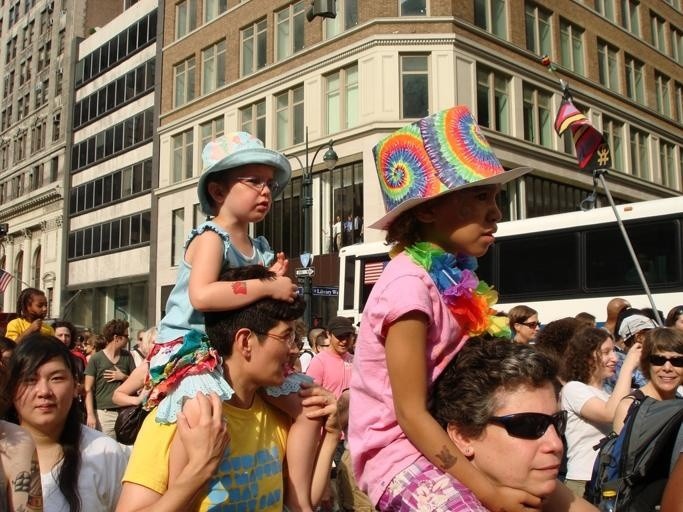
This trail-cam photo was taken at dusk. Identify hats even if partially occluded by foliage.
[367,102,533,231]
[618,314,658,343]
[197,132,292,214]
[327,315,357,338]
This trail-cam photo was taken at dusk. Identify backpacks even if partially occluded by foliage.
[582,394,682,512]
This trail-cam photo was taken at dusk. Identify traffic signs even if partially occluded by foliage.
[293,285,302,296]
[311,287,337,295]
[295,266,315,276]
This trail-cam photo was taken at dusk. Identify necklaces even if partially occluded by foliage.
[347,184,547,512]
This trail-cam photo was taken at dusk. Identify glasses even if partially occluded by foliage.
[485,409,569,439]
[255,330,298,347]
[117,335,129,339]
[520,320,541,329]
[222,174,280,192]
[648,354,682,368]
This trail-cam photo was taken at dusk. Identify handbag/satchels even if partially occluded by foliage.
[114,405,150,445]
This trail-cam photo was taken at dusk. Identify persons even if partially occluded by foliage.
[0,264,682,511]
[333,212,364,253]
[145,130,324,512]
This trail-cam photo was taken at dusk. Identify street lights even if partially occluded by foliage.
[283,126,338,339]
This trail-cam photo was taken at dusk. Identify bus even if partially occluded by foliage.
[335,195,682,354]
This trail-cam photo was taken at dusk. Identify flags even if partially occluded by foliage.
[554,88,614,172]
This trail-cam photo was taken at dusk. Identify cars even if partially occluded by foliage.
[40,317,106,345]
